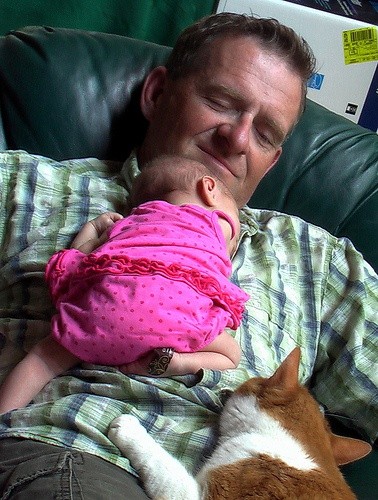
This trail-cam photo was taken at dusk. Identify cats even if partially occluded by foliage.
[107,345,372,500]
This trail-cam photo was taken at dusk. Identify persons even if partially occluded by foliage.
[0,11,378,500]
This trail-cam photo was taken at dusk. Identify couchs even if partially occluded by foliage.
[0,27,378,500]
